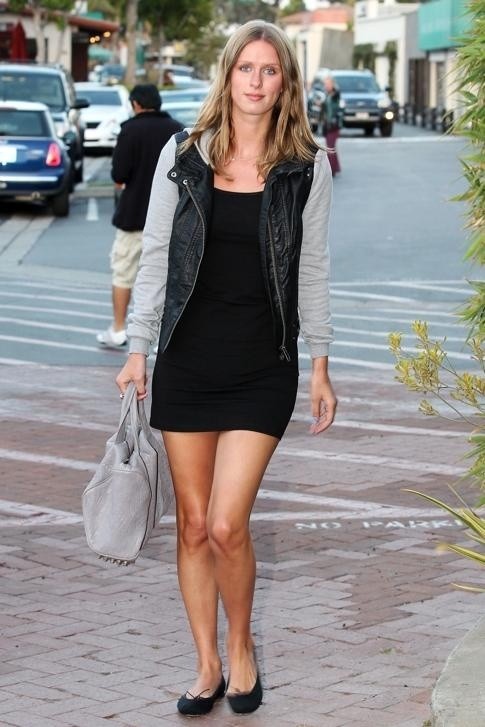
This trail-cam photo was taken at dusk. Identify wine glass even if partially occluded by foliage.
[119,392,125,399]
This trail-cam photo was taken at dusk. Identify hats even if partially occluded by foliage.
[88,58,215,131]
[73,77,129,152]
[1,100,76,220]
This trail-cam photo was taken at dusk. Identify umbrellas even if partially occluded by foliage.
[227,669,263,713]
[178,674,226,717]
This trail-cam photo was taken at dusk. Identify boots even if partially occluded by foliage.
[0,62,89,181]
[308,68,396,137]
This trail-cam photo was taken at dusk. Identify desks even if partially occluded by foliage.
[298,24,309,87]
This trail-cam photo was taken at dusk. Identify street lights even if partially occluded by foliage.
[81,379,176,567]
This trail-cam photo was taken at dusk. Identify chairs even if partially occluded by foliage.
[95,329,129,346]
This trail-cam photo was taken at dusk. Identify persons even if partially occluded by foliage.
[114,15,342,715]
[96,82,187,349]
[314,74,345,177]
